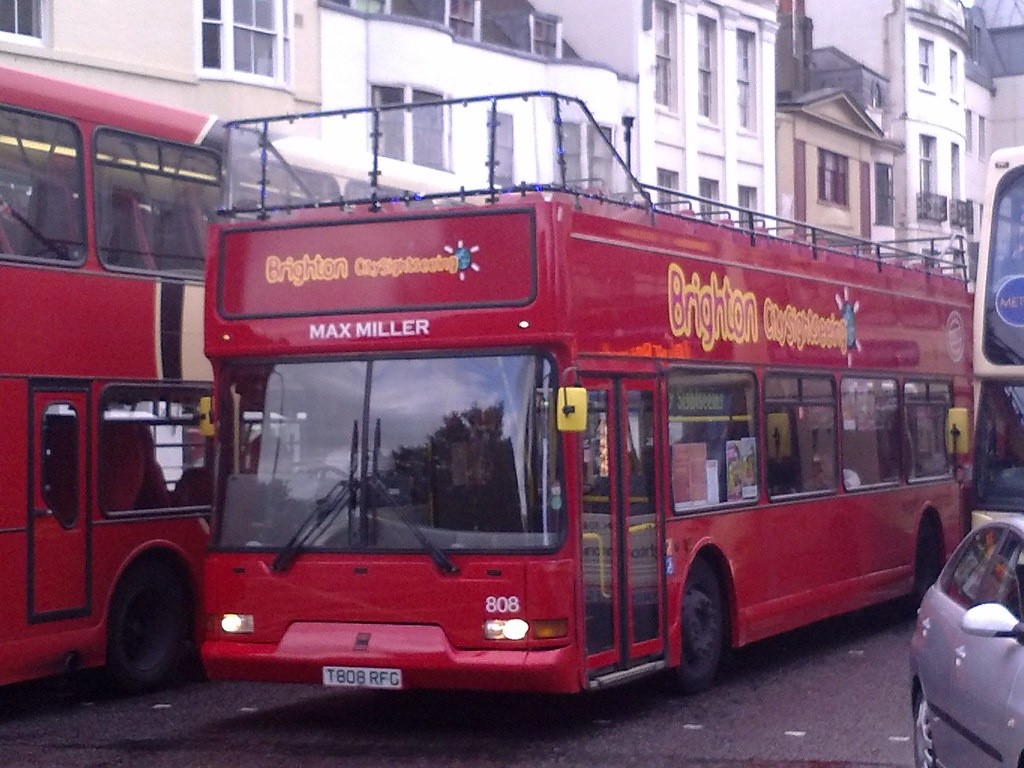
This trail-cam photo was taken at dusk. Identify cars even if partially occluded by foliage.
[906,518,1024,768]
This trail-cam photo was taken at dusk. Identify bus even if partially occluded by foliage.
[945,146,1023,567]
[195,91,979,699]
[0,68,285,695]
